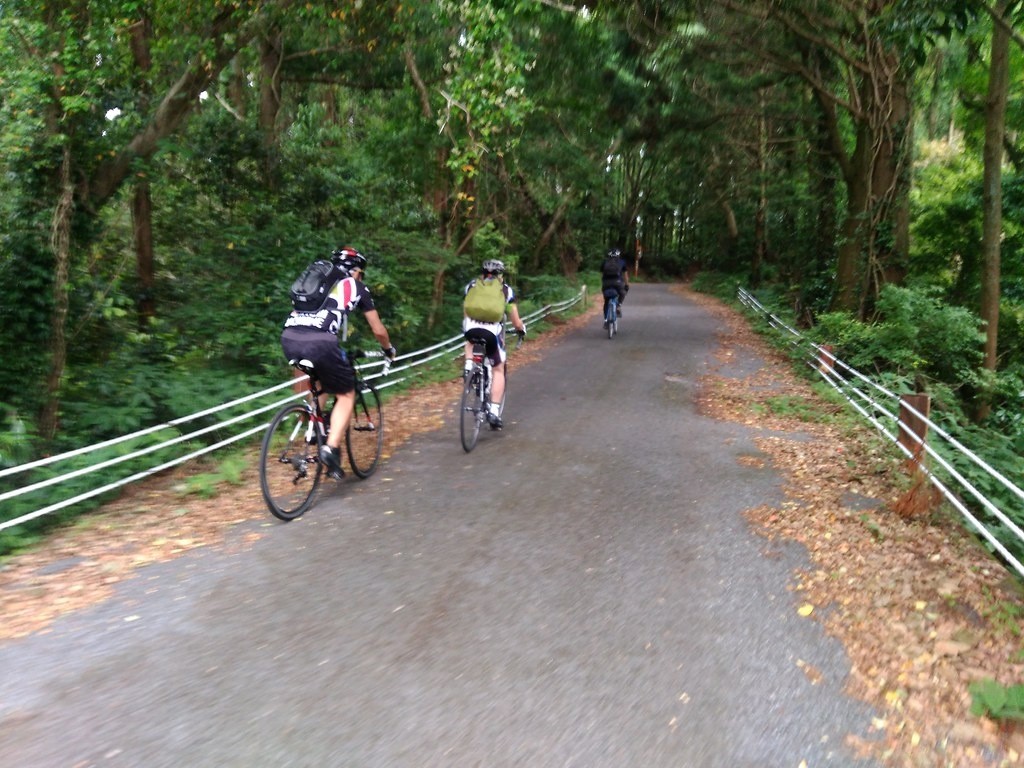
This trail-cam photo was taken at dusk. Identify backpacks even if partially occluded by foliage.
[292,260,356,341]
[464,278,505,322]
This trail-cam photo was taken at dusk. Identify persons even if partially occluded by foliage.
[464,259,526,428]
[281,246,396,479]
[601,250,628,330]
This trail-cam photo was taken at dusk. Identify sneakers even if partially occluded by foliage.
[319,445,345,480]
[487,413,503,428]
[305,431,316,445]
[464,370,477,384]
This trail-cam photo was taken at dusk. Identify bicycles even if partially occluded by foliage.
[259,347,396,521]
[605,286,627,339]
[459,328,526,451]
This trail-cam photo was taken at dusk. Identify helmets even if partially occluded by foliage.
[608,248,620,257]
[483,258,505,275]
[334,246,367,271]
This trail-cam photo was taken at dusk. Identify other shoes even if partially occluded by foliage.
[603,322,608,329]
[616,308,621,318]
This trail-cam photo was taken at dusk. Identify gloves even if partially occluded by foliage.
[516,325,526,336]
[624,285,629,292]
[381,344,396,358]
